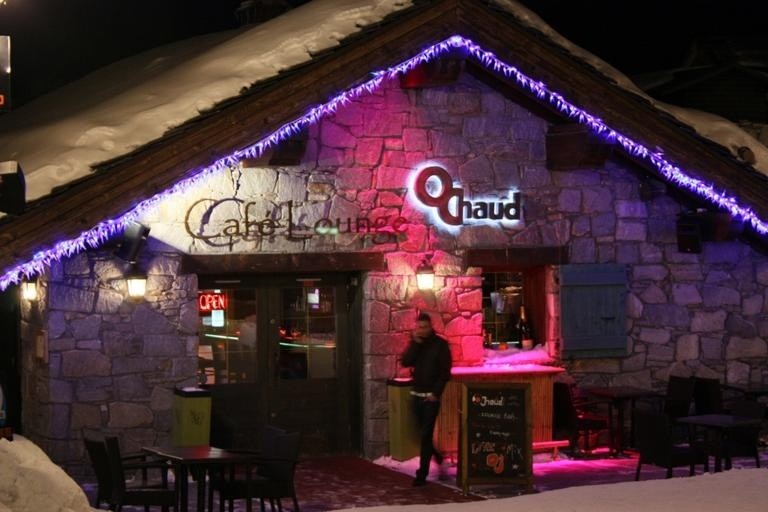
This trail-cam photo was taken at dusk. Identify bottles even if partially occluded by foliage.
[516,304,535,351]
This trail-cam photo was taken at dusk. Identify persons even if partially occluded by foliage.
[399,312,454,486]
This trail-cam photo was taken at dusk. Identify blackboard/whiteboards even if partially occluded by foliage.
[461,381,533,485]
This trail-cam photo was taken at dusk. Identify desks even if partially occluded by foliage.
[138,444,237,512]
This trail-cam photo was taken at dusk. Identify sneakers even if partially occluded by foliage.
[413,475,427,486]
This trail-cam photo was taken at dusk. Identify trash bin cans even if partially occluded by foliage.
[171,387,213,447]
[385,380,422,460]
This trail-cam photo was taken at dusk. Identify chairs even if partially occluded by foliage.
[553,374,765,480]
[80,426,182,512]
[209,436,301,511]
